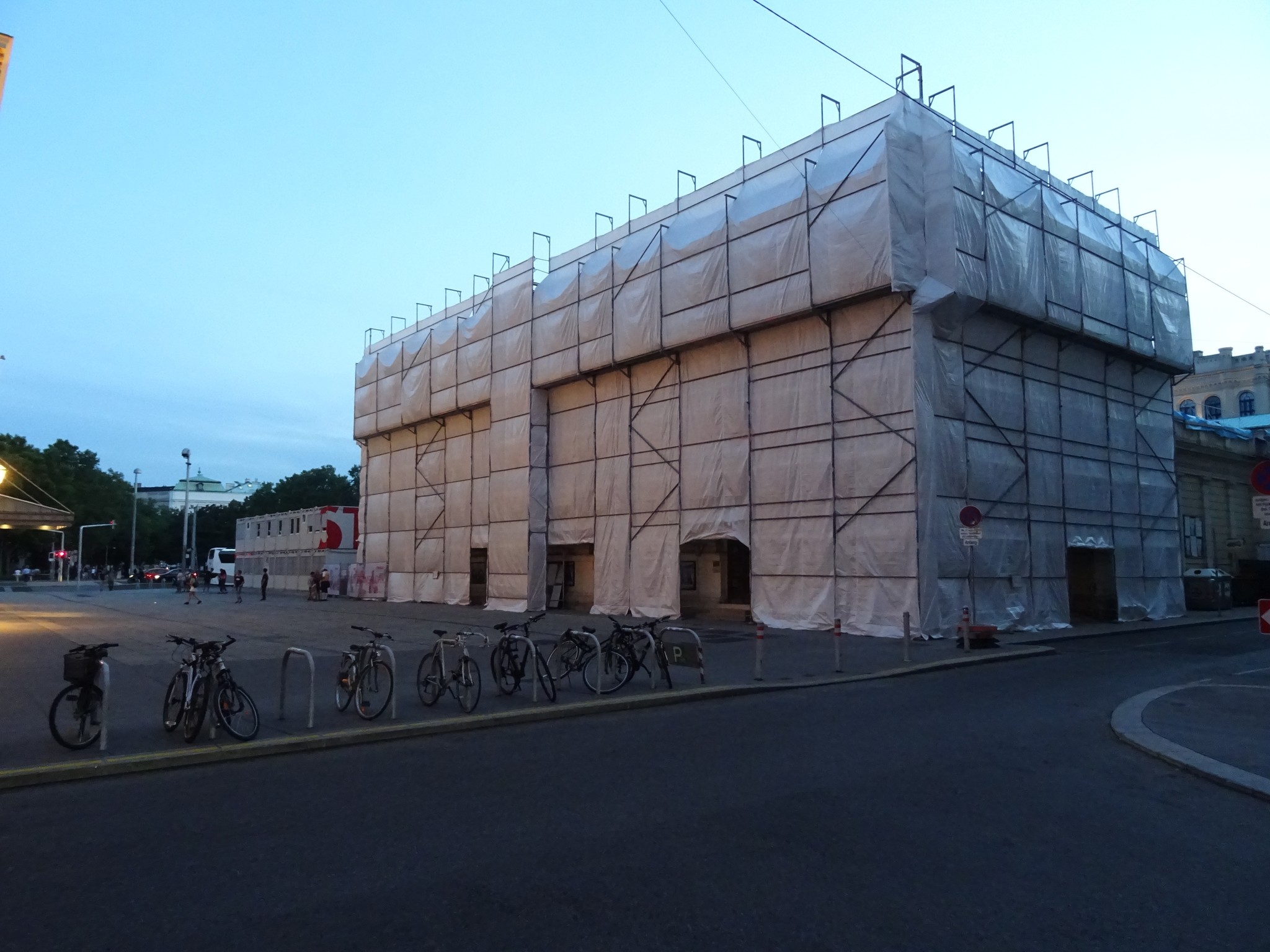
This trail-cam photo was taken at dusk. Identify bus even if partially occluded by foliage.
[207,548,236,586]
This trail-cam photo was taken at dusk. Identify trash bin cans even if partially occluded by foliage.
[1183,568,1234,611]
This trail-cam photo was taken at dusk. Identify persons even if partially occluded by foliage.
[82,561,329,605]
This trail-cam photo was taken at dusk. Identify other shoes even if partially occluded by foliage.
[197,601,201,604]
[235,601,238,603]
[308,598,327,601]
[184,602,189,604]
[260,598,265,600]
[238,599,242,603]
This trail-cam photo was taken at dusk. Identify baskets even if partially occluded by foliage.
[63,652,91,684]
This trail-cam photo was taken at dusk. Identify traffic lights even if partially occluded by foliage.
[60,552,64,557]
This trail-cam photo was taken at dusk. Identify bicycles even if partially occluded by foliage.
[415,628,491,715]
[491,611,559,705]
[160,632,261,743]
[335,625,393,722]
[46,641,120,751]
[545,614,675,695]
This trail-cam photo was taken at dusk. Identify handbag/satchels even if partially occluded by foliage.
[193,581,200,588]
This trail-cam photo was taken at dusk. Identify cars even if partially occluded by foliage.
[152,568,193,583]
[128,568,169,583]
